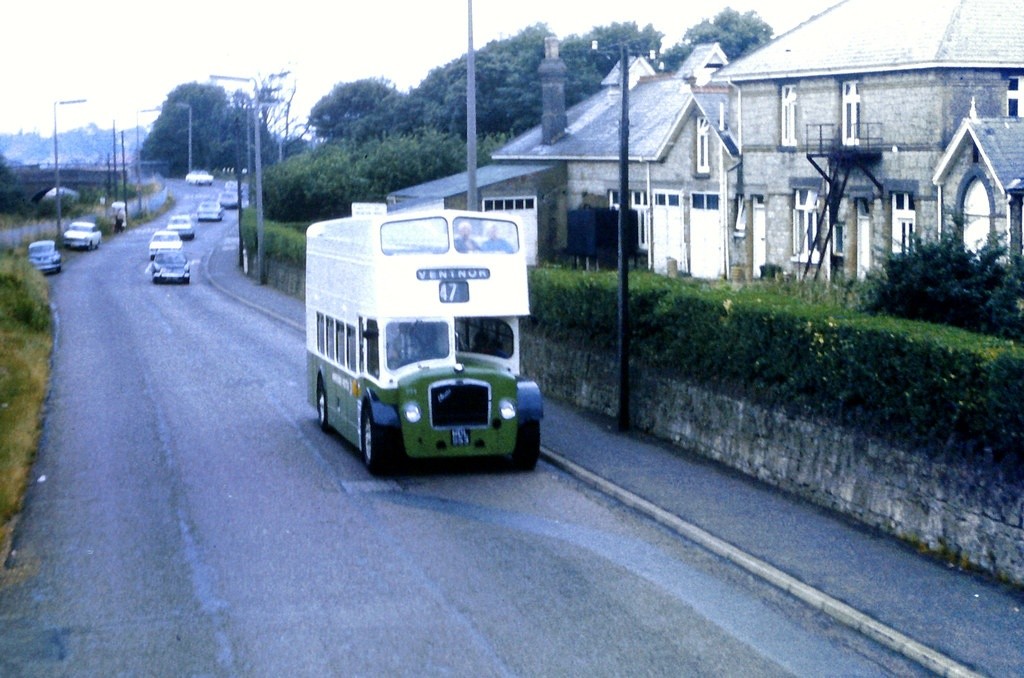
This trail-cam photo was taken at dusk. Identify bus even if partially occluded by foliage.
[305,208,544,472]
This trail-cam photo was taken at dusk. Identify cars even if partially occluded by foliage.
[186,170,213,186]
[64,222,101,251]
[149,181,238,284]
[28,240,63,273]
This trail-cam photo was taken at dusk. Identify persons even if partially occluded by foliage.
[455,223,514,254]
[471,332,507,359]
[113,207,126,234]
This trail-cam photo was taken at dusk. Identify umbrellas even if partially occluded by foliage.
[112,201,125,209]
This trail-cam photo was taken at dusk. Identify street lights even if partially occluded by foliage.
[210,75,264,285]
[54,99,86,234]
[591,39,656,426]
[136,109,156,217]
[177,103,192,173]
